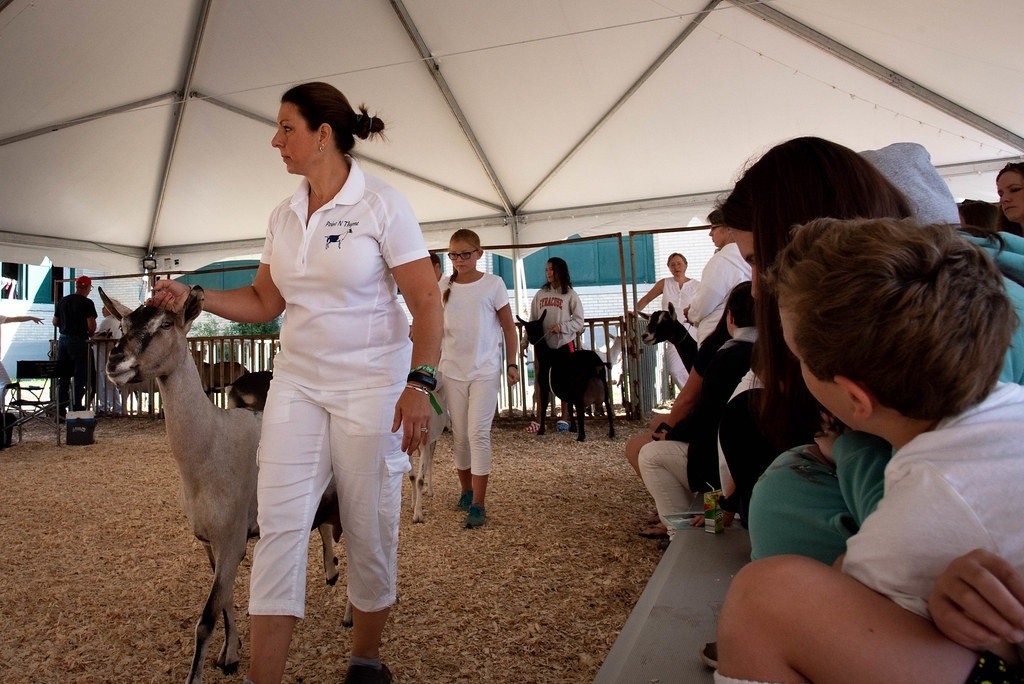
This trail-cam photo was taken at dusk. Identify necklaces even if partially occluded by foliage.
[311,190,324,206]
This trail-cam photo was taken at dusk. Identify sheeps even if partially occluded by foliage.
[401,369,446,523]
[637,301,698,373]
[516,309,615,440]
[99,284,352,684]
[189,348,275,411]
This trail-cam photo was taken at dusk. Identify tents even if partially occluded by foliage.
[0,0,1024,419]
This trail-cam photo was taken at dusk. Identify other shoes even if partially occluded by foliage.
[555,420,570,432]
[640,512,668,538]
[343,663,393,684]
[465,503,487,528]
[523,422,548,433]
[700,641,717,670]
[458,490,474,510]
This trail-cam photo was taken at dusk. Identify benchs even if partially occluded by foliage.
[583,493,750,684]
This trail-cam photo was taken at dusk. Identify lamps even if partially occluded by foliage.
[143,257,156,272]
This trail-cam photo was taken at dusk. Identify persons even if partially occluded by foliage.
[0,315,45,413]
[54,277,98,424]
[413,252,450,433]
[520,258,585,433]
[93,306,121,417]
[624,133,1024,684]
[152,83,445,684]
[438,229,519,529]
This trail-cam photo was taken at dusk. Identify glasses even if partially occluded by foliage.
[448,249,478,261]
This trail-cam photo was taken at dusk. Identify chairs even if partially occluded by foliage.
[2,360,76,448]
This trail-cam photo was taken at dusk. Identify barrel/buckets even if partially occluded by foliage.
[65,419,96,446]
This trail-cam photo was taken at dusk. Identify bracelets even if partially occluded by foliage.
[507,364,518,372]
[188,284,193,290]
[405,364,443,416]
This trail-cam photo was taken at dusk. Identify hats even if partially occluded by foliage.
[76,276,94,288]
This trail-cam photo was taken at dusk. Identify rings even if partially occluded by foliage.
[421,428,427,432]
